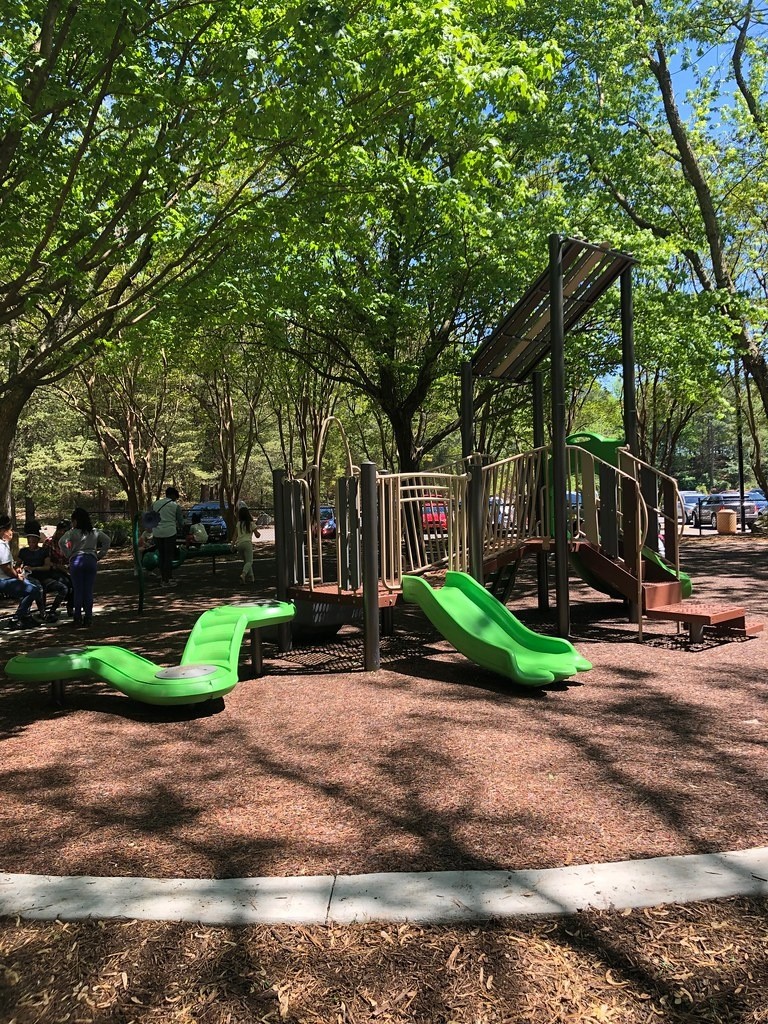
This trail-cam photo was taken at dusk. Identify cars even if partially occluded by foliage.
[566,492,583,534]
[747,488,768,516]
[183,501,235,543]
[421,494,452,534]
[311,506,337,539]
[661,491,707,525]
[488,494,513,536]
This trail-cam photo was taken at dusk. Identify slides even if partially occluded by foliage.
[402,573,591,688]
[639,548,691,598]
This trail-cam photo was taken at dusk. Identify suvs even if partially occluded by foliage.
[691,490,758,530]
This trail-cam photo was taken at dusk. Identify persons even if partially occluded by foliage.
[323,508,334,530]
[138,487,208,587]
[0,508,111,630]
[231,507,261,584]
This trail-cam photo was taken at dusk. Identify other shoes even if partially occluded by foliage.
[247,574,255,582]
[160,580,178,588]
[67,612,75,617]
[71,620,93,628]
[40,609,58,623]
[240,575,245,585]
[7,615,41,630]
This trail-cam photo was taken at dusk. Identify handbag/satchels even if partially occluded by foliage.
[142,510,161,528]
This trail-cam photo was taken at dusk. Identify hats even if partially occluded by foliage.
[0,515,11,528]
[24,532,41,539]
